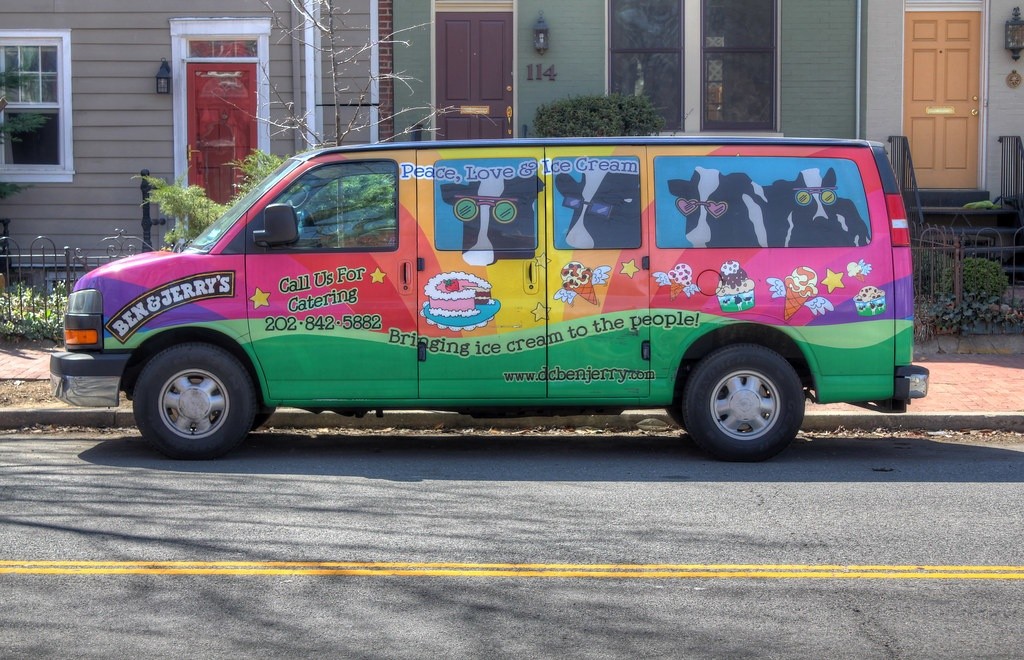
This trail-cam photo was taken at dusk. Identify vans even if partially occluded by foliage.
[50,134,928,461]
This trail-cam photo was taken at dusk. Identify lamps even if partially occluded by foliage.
[1005,6,1024,62]
[532,10,550,55]
[155,57,170,94]
